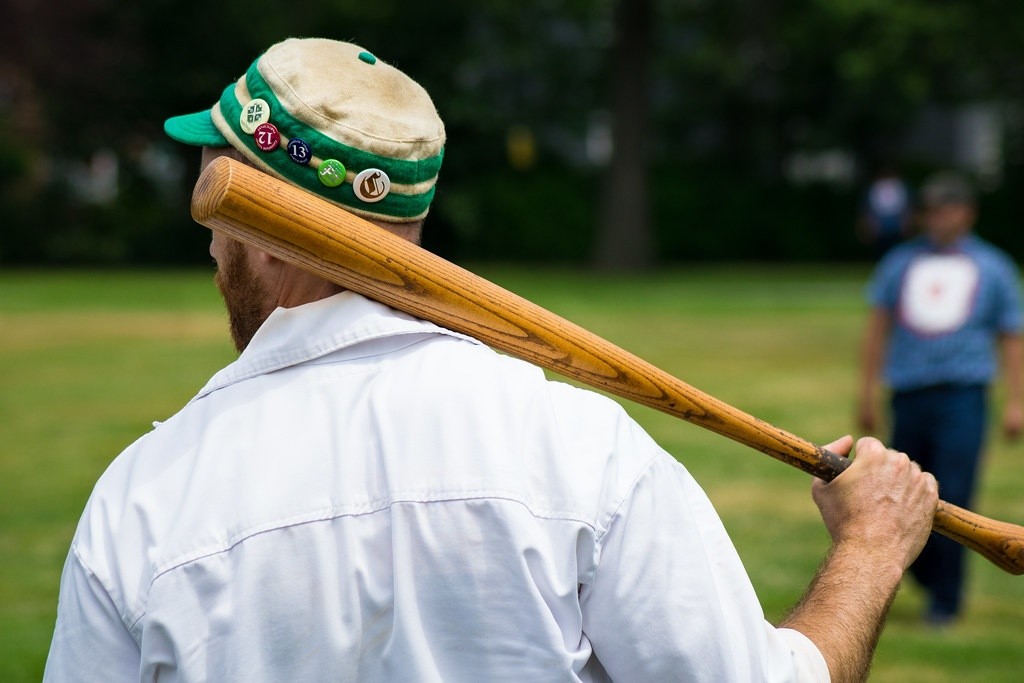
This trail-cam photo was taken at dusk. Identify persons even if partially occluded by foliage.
[856,171,1023,625]
[36,37,940,683]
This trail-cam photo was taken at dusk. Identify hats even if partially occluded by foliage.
[163,37,446,223]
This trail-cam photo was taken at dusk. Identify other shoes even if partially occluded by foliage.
[925,590,962,623]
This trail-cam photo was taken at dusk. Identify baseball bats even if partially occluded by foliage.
[190,156,1024,582]
